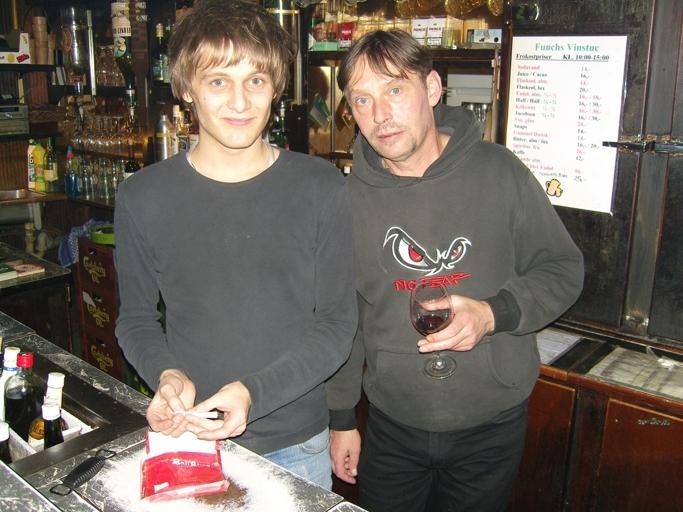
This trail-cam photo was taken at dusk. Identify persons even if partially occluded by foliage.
[328,28,585,510]
[112,1,355,491]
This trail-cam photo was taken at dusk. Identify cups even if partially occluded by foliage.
[31,13,49,65]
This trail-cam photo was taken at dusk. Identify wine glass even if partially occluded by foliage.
[93,45,126,90]
[408,282,462,382]
[65,110,147,154]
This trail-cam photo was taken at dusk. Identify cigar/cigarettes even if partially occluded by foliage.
[181,410,218,419]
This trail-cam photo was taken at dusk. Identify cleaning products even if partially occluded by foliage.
[27,139,45,193]
[65,147,78,197]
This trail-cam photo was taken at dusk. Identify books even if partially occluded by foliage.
[0,253,46,282]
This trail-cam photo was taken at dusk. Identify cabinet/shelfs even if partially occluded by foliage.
[48,63,136,213]
[577,388,682,510]
[515,378,575,512]
[0,62,62,140]
[504,1,683,348]
[305,49,500,164]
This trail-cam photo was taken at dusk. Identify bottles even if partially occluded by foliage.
[58,1,90,100]
[150,12,172,83]
[23,137,67,196]
[269,101,291,151]
[108,0,138,109]
[64,150,141,200]
[152,102,199,162]
[0,344,68,464]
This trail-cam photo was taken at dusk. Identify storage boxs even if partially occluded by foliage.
[77,241,121,378]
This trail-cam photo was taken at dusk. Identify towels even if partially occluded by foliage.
[54,219,114,268]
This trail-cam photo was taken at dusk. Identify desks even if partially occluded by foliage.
[1,312,33,343]
[0,333,369,511]
[0,241,73,352]
